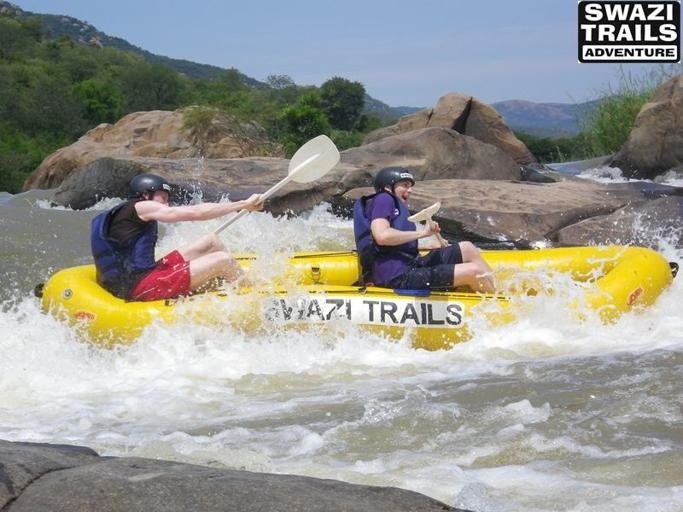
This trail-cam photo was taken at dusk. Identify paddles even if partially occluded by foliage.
[407,201,447,247]
[214,134,340,234]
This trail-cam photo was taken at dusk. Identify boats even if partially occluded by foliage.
[32,246,680,357]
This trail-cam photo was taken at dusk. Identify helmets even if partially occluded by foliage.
[127,173,170,197]
[374,166,416,192]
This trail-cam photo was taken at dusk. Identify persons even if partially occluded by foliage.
[89,173,264,302]
[352,165,495,292]
